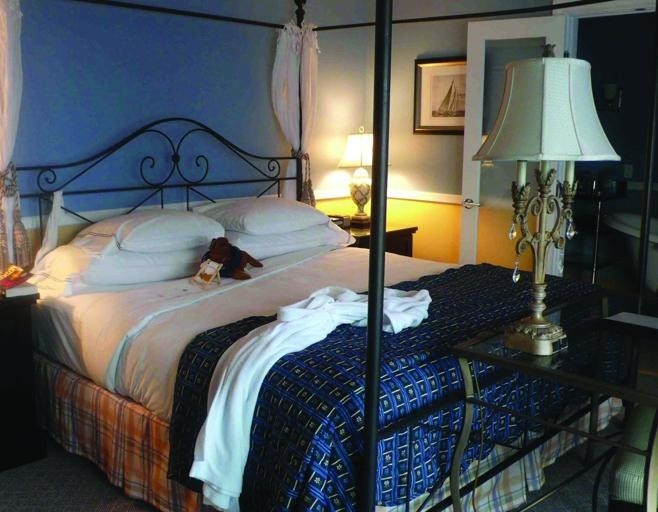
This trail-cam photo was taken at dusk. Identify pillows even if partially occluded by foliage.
[24,196,358,299]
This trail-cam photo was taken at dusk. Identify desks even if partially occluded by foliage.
[448,290,657,512]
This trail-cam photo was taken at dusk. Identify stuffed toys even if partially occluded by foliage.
[200,236,264,282]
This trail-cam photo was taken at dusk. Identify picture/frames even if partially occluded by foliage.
[412,56,465,136]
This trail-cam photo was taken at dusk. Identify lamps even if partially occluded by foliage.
[469,44,624,358]
[601,83,624,113]
[334,123,394,230]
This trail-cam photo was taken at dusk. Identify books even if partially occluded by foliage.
[0,262,39,298]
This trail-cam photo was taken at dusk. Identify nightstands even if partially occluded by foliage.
[340,222,418,258]
[0,292,47,473]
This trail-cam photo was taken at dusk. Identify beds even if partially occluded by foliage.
[8,116,658,511]
